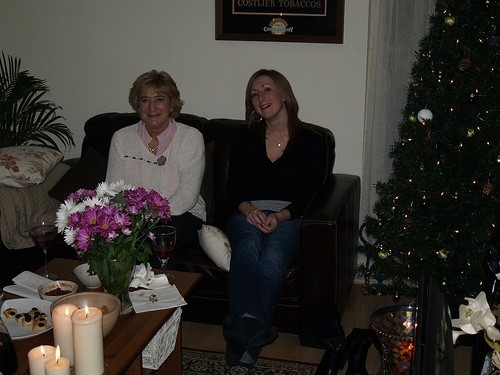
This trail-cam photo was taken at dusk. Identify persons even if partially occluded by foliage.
[106,69,207,269]
[217,69,322,367]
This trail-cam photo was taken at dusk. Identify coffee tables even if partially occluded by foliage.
[0,257,202,375]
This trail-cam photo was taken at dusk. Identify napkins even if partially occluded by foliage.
[129,274,187,313]
[2,270,54,300]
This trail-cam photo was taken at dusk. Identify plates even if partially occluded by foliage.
[0,299,54,342]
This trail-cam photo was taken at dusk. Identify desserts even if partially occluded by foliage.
[3,307,48,331]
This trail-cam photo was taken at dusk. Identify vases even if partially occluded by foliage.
[370,305,416,375]
[95,260,136,315]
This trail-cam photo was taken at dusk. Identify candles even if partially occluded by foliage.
[71,304,104,375]
[28,345,55,375]
[45,344,71,375]
[52,304,78,366]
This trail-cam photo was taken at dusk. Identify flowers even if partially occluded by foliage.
[55,179,172,310]
[451,291,500,375]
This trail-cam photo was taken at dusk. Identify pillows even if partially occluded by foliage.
[197,225,232,271]
[0,146,64,187]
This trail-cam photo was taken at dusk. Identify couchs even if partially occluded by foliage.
[0,113,360,348]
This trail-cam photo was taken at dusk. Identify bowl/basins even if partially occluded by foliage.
[38,280,77,301]
[73,263,102,290]
[50,292,122,340]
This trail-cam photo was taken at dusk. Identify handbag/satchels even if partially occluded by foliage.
[314,328,383,374]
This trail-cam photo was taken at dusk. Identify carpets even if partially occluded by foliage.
[182,346,319,375]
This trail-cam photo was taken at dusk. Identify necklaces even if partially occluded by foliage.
[270,131,288,146]
[149,145,157,152]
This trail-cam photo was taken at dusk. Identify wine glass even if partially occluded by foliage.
[149,226,176,283]
[26,221,61,281]
